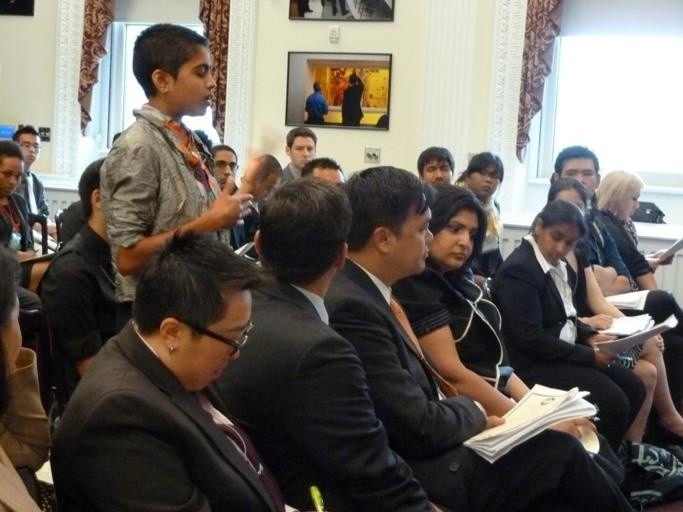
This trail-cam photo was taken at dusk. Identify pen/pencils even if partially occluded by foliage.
[309,485,324,512]
[248,200,259,215]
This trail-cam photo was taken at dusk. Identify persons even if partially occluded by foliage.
[342,70,364,126]
[305,81,328,124]
[0,123,682,512]
[99,25,253,328]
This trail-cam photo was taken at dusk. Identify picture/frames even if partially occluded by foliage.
[284,51,393,132]
[289,0,394,22]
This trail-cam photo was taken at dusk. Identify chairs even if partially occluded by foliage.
[27,214,49,255]
[55,208,68,245]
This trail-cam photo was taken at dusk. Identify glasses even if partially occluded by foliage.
[21,142,40,150]
[170,314,256,358]
[215,160,239,171]
